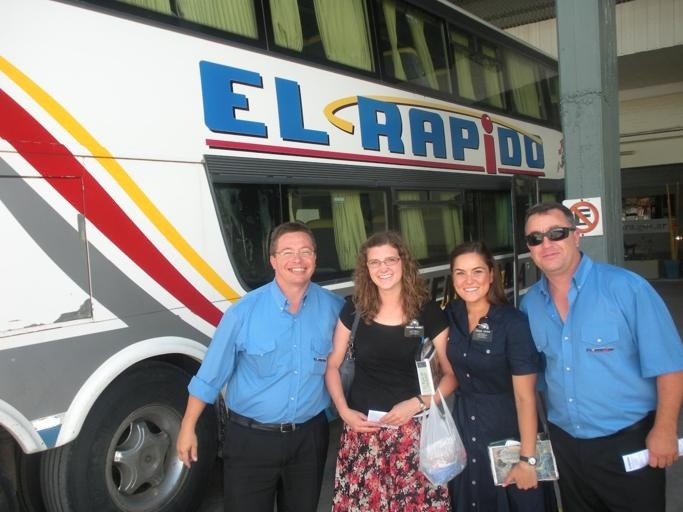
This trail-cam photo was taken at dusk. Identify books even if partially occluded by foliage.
[487,439,560,487]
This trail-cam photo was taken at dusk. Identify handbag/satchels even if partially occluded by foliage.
[328,341,355,417]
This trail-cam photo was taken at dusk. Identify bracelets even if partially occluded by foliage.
[415,395,426,410]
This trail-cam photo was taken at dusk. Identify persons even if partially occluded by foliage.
[174,220,351,512]
[438,239,550,511]
[510,199,683,511]
[322,228,460,511]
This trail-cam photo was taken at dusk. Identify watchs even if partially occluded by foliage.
[519,455,537,465]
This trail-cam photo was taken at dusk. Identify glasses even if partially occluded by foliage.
[367,257,400,269]
[279,249,314,260]
[524,226,575,246]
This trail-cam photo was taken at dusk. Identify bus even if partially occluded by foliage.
[1,0,570,511]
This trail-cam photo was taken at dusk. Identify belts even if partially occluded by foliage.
[229,411,325,433]
